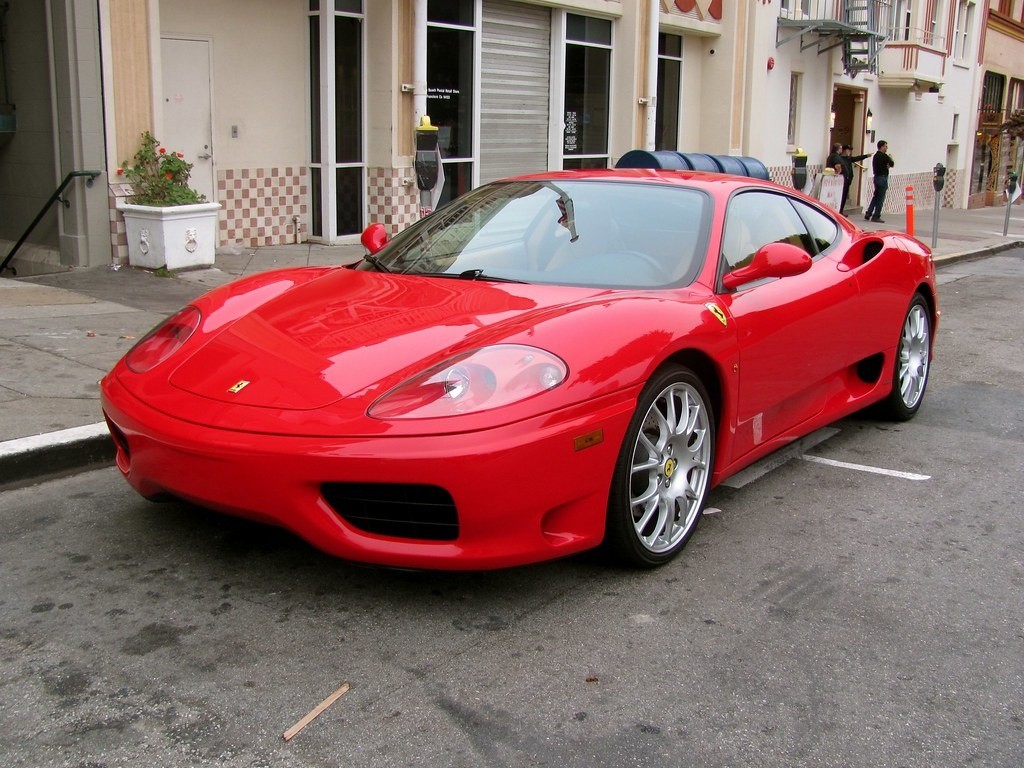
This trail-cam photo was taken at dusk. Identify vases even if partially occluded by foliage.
[116,203,222,272]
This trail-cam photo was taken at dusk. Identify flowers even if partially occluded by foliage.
[117,130,209,207]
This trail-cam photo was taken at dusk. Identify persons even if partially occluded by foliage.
[864,140,894,223]
[827,143,874,217]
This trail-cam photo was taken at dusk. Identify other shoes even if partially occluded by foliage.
[871,217,885,223]
[863,214,868,221]
[844,214,848,217]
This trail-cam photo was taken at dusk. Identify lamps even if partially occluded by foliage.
[866,108,873,133]
[830,104,837,130]
[930,84,940,94]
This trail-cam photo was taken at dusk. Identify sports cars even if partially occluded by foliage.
[99,167,938,567]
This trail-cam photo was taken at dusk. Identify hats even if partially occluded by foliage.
[842,144,853,150]
[832,143,841,150]
